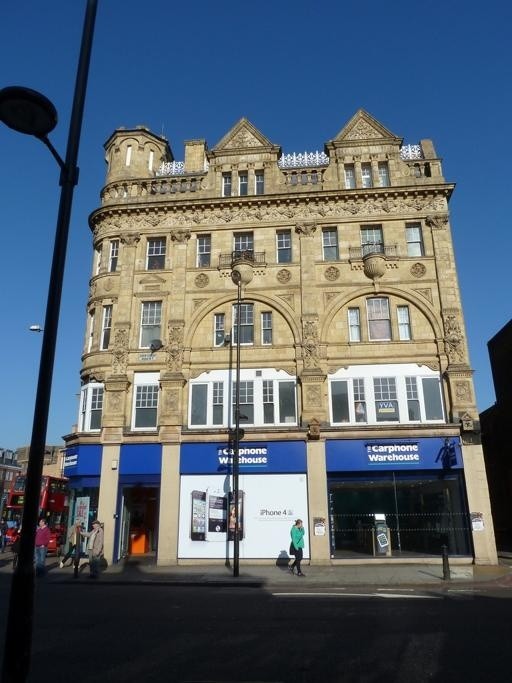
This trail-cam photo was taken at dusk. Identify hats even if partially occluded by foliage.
[92,520,100,523]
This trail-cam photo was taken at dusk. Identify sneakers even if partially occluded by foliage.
[60,561,63,568]
[73,565,80,568]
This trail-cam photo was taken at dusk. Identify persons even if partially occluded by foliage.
[59,519,80,568]
[12,528,21,570]
[35,519,51,576]
[0,516,8,553]
[80,520,103,579]
[288,519,305,576]
[229,506,238,528]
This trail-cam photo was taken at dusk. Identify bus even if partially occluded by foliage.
[0,471,68,555]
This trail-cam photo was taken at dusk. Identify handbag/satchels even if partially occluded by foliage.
[290,546,297,554]
[92,556,107,571]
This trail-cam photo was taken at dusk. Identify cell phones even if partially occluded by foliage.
[227,490,245,541]
[189,490,206,541]
[205,487,230,541]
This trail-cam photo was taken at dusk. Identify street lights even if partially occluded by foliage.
[0,82,89,682]
[227,270,249,577]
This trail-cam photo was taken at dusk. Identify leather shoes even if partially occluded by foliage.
[298,572,305,575]
